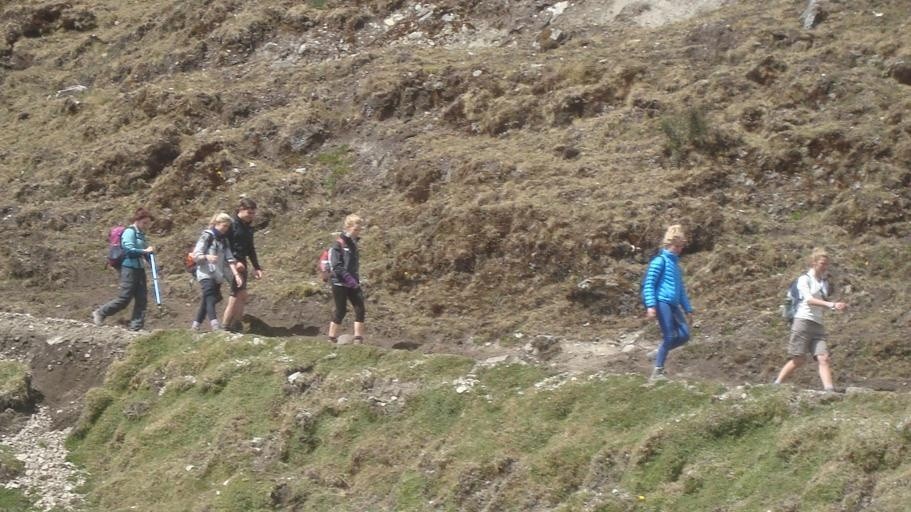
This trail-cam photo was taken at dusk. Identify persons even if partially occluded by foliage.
[92,206,155,333]
[774,249,851,390]
[220,197,263,334]
[643,224,693,383]
[327,213,365,344]
[189,212,244,334]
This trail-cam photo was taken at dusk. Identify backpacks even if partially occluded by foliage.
[782,274,822,321]
[320,237,344,273]
[108,224,136,266]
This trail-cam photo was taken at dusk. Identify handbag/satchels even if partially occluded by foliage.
[184,254,197,273]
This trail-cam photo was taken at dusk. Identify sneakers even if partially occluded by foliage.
[138,327,151,332]
[328,337,337,342]
[647,350,659,361]
[649,368,671,382]
[826,388,845,394]
[192,320,242,335]
[94,309,105,325]
[353,336,362,344]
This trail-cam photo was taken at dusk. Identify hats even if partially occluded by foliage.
[135,207,154,221]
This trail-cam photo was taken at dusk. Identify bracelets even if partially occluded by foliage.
[831,304,835,311]
[144,248,147,254]
[234,272,240,276]
